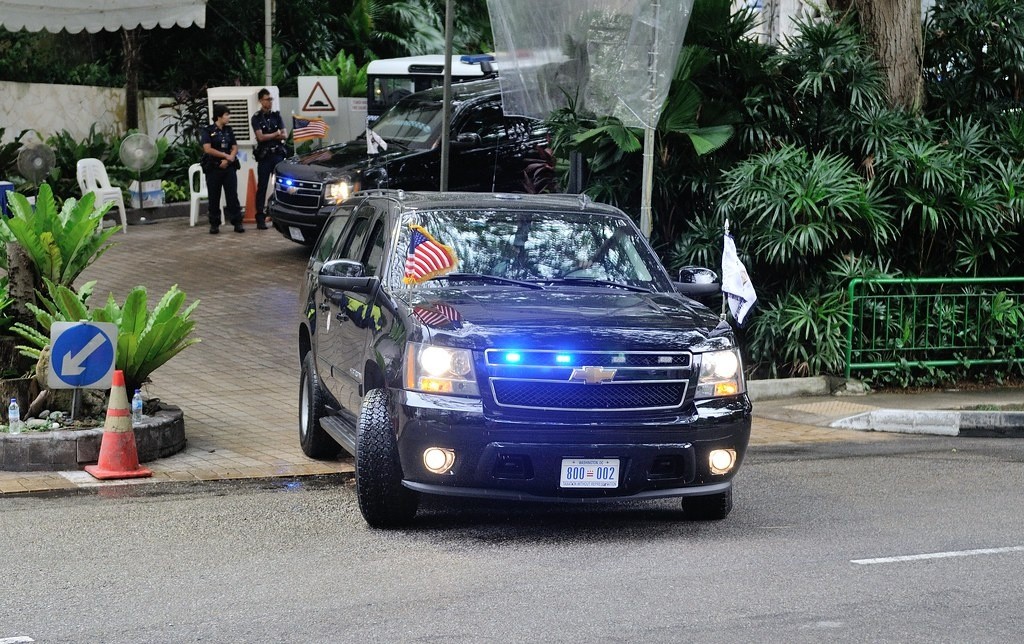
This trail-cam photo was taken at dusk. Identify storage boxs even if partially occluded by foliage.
[128,179,163,209]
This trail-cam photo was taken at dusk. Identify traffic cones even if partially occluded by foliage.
[81,370,165,482]
[241,170,274,220]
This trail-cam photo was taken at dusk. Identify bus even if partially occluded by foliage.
[365,49,587,138]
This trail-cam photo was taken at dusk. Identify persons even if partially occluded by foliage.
[251,88,286,228]
[201,105,245,234]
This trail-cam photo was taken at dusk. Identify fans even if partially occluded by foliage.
[119,133,159,225]
[17,141,56,206]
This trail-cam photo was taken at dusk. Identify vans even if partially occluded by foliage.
[268,76,562,248]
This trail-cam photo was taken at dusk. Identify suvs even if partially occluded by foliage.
[294,189,754,529]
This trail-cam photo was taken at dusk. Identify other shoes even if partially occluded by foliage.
[258,219,268,229]
[210,225,219,233]
[234,224,244,232]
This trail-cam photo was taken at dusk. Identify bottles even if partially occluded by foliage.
[132,388,142,426]
[8,399,20,435]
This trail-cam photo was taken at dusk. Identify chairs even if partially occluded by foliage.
[188,162,227,227]
[76,158,127,236]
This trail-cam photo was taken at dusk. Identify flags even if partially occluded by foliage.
[720,234,758,325]
[415,301,462,329]
[402,226,458,285]
[291,114,325,142]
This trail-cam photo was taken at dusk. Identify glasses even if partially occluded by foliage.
[263,98,272,101]
